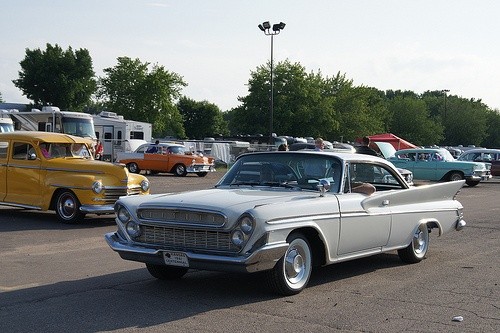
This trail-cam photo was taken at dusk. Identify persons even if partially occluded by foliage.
[94,140,104,160]
[278,136,377,194]
[145,140,161,174]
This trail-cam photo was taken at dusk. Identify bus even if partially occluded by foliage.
[0,106,98,159]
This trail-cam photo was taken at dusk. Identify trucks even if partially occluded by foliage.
[93,111,152,163]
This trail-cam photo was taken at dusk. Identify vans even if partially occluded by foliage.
[457,149,500,177]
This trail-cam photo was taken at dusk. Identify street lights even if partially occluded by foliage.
[258,21,286,137]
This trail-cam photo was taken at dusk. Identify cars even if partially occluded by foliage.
[0,131,150,225]
[104,150,467,295]
[374,142,493,187]
[117,139,216,177]
